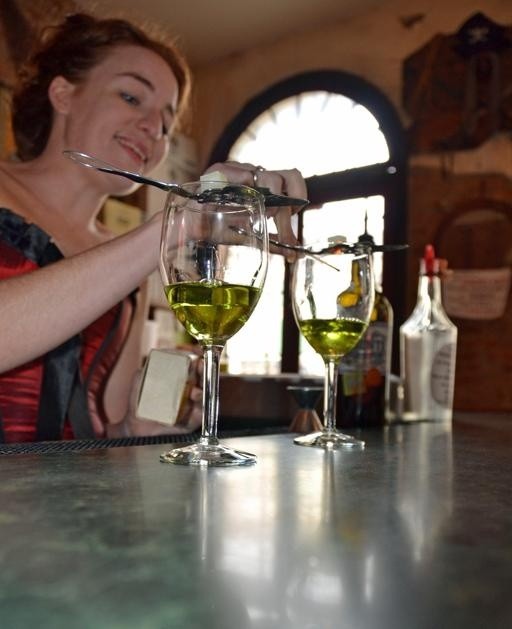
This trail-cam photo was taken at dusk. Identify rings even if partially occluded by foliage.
[249,165,266,189]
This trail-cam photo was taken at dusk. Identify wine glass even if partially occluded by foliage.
[289,243,376,451]
[156,180,271,468]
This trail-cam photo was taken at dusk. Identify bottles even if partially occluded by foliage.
[397,245,459,423]
[331,233,394,432]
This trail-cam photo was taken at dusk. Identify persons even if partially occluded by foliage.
[1,9,308,443]
